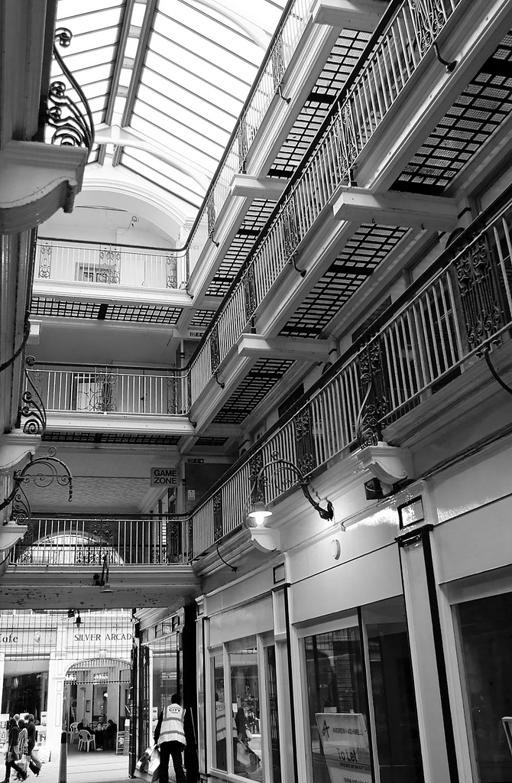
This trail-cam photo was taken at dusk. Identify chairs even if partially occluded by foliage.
[69,722,124,752]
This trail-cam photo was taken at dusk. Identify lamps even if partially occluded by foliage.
[93,554,115,594]
[73,610,83,628]
[250,449,334,527]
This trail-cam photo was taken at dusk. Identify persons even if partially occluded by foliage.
[0,711,43,783]
[215,691,238,772]
[233,700,250,744]
[76,715,117,753]
[153,692,189,782]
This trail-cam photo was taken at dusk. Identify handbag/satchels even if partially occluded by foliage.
[236,741,261,774]
[134,745,161,783]
